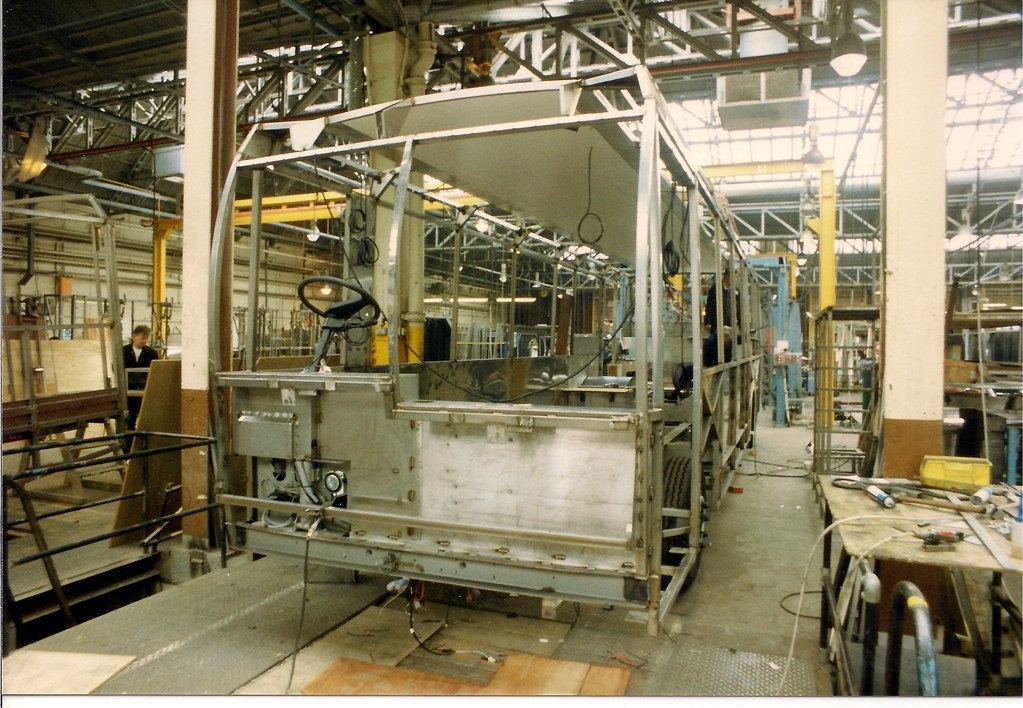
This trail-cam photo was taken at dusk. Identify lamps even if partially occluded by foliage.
[830,1,870,79]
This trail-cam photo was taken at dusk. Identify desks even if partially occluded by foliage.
[812,473,1023,652]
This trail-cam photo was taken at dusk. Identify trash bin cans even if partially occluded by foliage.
[942,423,963,456]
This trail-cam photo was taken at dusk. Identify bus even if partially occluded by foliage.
[207,64,764,635]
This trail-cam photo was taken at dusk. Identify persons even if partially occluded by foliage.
[112,325,159,455]
[858,350,866,359]
[705,267,741,338]
[704,325,741,368]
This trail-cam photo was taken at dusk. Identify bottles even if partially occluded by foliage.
[1010,491,1023,559]
[864,484,896,508]
[971,487,992,506]
